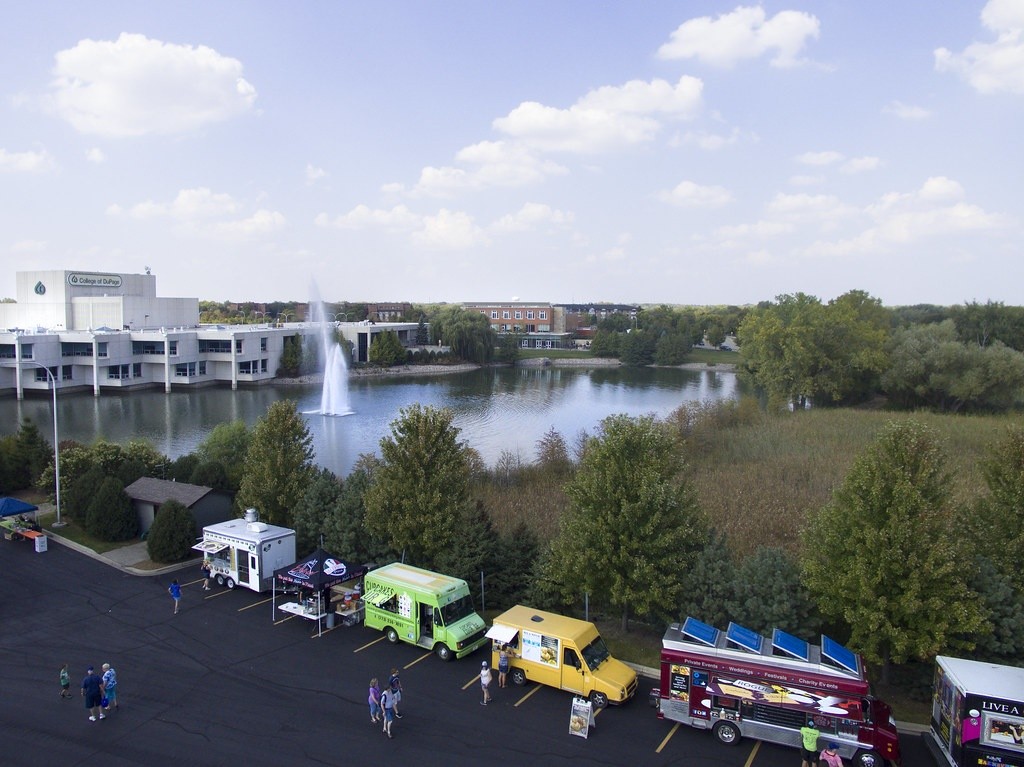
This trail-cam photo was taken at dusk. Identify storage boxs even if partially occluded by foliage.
[343,619,356,626]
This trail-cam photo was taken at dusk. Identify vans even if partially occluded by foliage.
[484,604,639,709]
[363,562,489,662]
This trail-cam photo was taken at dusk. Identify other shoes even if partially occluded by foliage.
[395,713,402,718]
[203,586,205,588]
[205,587,211,590]
[59,693,65,697]
[371,719,377,723]
[376,717,381,721]
[480,701,487,705]
[502,685,508,688]
[383,729,387,732]
[66,695,72,698]
[387,733,392,738]
[499,685,502,687]
[487,698,492,701]
[174,611,178,614]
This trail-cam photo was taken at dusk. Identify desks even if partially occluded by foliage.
[335,606,365,623]
[277,602,328,631]
[0,519,42,541]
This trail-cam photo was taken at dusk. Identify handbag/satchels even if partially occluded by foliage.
[101,695,108,707]
[378,694,386,708]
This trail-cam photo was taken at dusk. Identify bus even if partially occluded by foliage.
[918,656,1024,767]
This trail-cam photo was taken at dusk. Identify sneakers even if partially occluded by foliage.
[99,714,105,719]
[89,716,96,721]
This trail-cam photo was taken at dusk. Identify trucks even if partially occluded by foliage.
[191,518,297,593]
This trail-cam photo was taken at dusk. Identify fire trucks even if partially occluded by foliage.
[651,617,901,766]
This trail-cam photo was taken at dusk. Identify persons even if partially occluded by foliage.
[799,719,878,767]
[59,663,72,699]
[480,661,492,705]
[81,663,120,722]
[200,560,212,591]
[498,643,514,688]
[368,668,404,738]
[168,579,182,613]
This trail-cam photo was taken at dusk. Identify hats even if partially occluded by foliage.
[87,665,94,671]
[482,661,487,667]
